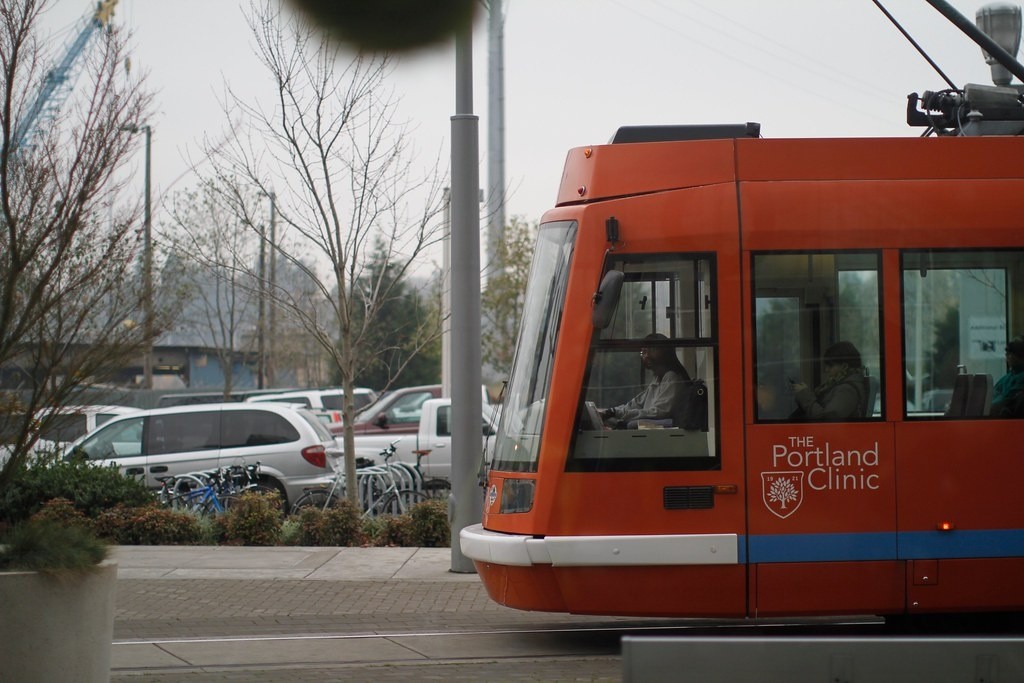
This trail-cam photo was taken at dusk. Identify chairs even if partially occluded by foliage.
[968,372,992,416]
[859,375,879,416]
[943,374,967,417]
[627,380,702,431]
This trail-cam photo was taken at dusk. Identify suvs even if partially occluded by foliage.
[39,402,345,511]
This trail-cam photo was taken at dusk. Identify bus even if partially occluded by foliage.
[451,80,1024,653]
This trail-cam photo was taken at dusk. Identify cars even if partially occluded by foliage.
[243,370,551,493]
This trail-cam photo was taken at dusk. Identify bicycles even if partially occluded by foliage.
[124,441,449,526]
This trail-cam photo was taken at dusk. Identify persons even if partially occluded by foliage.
[791,340,869,420]
[991,335,1024,419]
[599,333,691,430]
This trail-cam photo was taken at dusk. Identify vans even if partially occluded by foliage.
[23,397,146,481]
